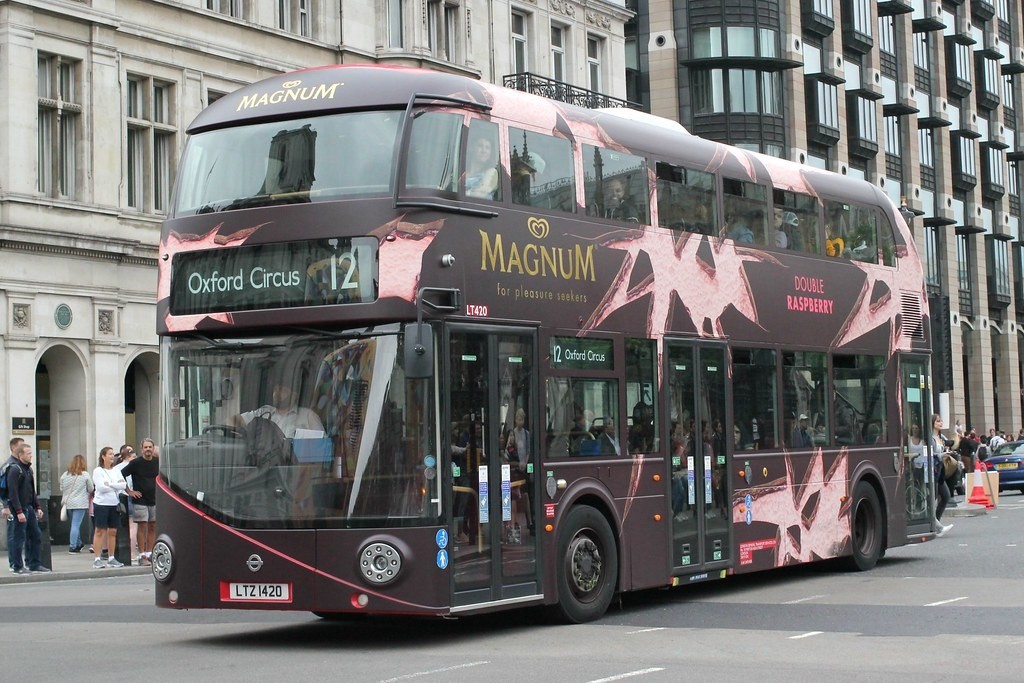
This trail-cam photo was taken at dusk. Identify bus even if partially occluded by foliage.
[151,63,938,624]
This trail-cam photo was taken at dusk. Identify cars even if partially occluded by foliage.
[983,439,1024,494]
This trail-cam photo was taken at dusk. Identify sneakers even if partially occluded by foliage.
[107,558,124,567]
[93,559,105,568]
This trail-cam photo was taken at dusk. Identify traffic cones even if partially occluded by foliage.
[967,460,990,509]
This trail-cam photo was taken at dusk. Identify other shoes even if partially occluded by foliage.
[148,554,152,562]
[9,564,15,571]
[139,556,151,566]
[89,544,94,553]
[25,564,30,569]
[100,551,109,560]
[69,544,84,554]
[13,567,32,575]
[936,524,953,538]
[29,565,51,573]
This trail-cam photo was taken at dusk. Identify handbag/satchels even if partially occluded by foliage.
[116,503,126,514]
[60,504,68,521]
[943,455,958,480]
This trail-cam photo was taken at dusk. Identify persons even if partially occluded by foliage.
[511,151,546,206]
[231,376,328,475]
[929,516,954,538]
[656,176,895,268]
[587,173,644,224]
[449,402,926,545]
[932,413,950,522]
[462,136,499,201]
[7,443,51,574]
[59,454,95,555]
[954,419,1024,495]
[89,438,159,568]
[0,438,26,572]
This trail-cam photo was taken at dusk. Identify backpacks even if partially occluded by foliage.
[246,412,299,466]
[0,462,33,502]
[978,447,987,461]
